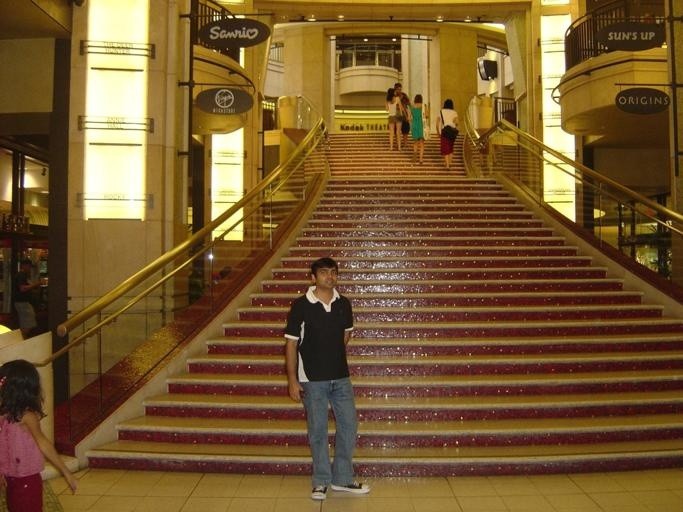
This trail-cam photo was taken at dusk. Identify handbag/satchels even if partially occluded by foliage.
[422,127,431,140]
[402,120,409,133]
[441,125,458,141]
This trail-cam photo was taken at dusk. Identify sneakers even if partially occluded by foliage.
[330,482,371,494]
[311,487,328,500]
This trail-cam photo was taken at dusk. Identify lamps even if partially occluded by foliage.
[305,12,316,22]
[435,15,444,22]
[464,16,472,22]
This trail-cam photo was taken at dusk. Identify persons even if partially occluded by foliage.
[13,258,45,335]
[437,98,459,168]
[394,83,411,147]
[385,88,407,151]
[284,257,370,500]
[407,94,429,163]
[0,359,76,512]
